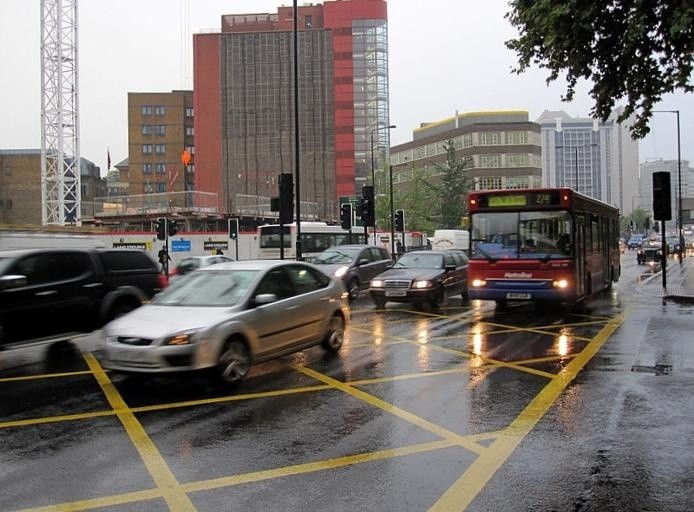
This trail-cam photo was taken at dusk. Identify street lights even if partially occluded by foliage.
[371,125,395,246]
[556,144,599,192]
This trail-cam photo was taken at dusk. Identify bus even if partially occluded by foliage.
[256,222,370,263]
[466,189,622,313]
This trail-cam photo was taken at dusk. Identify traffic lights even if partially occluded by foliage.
[341,204,351,229]
[653,222,659,232]
[652,172,673,222]
[228,218,239,239]
[356,187,376,226]
[644,217,650,229]
[270,173,294,225]
[395,211,404,233]
[155,216,178,240]
[630,221,633,231]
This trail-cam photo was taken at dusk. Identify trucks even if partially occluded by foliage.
[432,231,470,253]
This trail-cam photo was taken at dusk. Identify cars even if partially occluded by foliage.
[367,251,468,311]
[617,238,627,255]
[628,234,645,251]
[166,257,236,291]
[666,235,685,255]
[308,244,393,302]
[101,260,349,389]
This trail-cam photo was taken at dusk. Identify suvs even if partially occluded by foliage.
[637,238,668,264]
[0,248,164,382]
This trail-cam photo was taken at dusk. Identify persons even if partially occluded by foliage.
[158,244,171,273]
[216,249,223,255]
